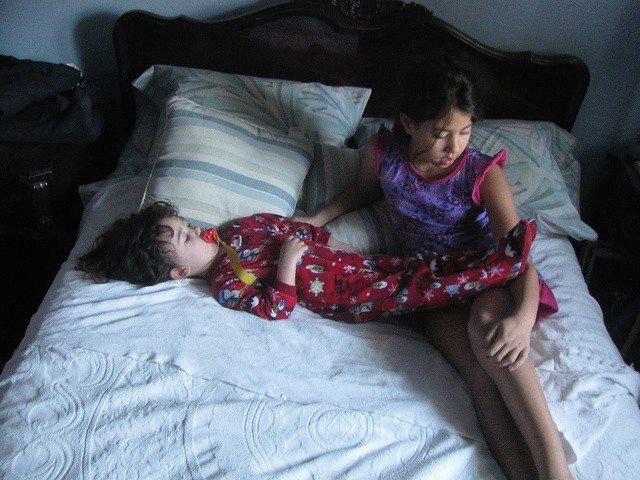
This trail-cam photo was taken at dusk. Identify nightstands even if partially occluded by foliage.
[0,90,122,367]
[576,174,640,367]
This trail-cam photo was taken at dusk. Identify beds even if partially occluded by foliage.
[0,1,640,479]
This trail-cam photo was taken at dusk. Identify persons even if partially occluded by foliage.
[290,58,573,479]
[75,201,538,324]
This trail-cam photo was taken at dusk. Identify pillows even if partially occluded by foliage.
[355,117,599,242]
[136,94,316,231]
[298,142,414,254]
[130,65,374,148]
